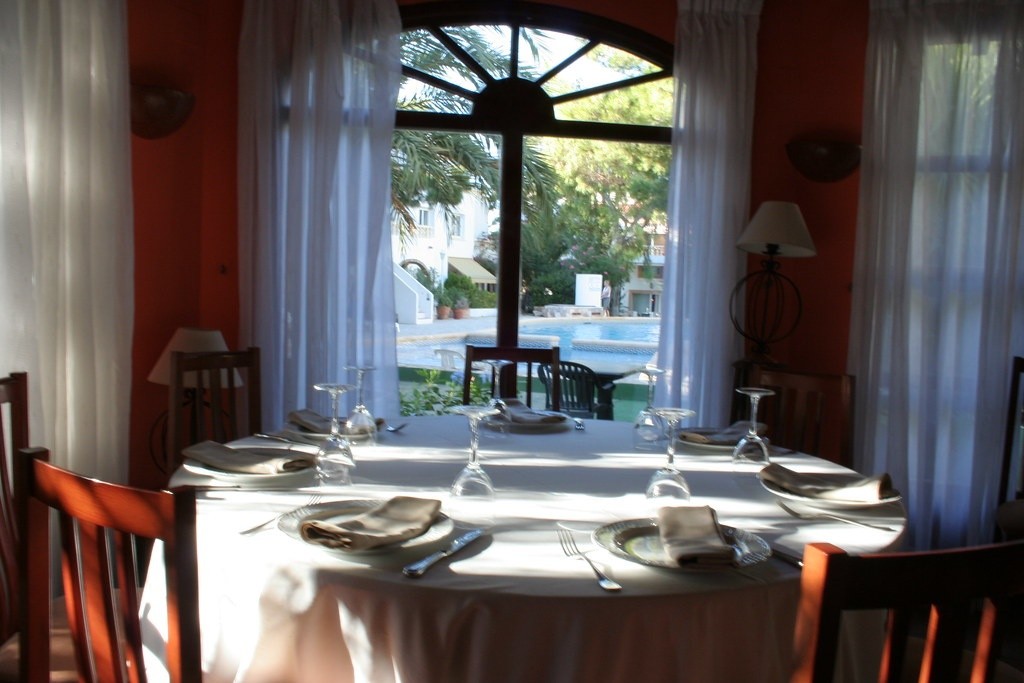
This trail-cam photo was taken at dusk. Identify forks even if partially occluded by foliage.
[777,501,894,534]
[557,529,622,590]
[383,421,409,433]
[240,494,322,535]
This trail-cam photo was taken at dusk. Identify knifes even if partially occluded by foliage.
[402,530,485,576]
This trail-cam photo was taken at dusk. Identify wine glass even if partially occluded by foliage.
[635,367,664,448]
[645,408,697,512]
[447,404,500,515]
[477,357,514,438]
[342,363,380,450]
[734,387,776,470]
[313,383,359,488]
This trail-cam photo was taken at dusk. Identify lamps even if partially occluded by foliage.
[728,202,816,367]
[149,327,243,478]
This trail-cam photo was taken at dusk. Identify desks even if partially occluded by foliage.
[546,360,642,421]
[172,411,917,683]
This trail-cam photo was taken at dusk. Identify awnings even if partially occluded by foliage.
[448,256,496,284]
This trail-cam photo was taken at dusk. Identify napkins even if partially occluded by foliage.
[288,408,384,435]
[659,506,736,571]
[760,463,892,500]
[301,496,441,552]
[496,399,567,423]
[181,439,315,474]
[677,421,768,443]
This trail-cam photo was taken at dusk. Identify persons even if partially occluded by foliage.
[601,280,611,317]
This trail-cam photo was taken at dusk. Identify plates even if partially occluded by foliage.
[278,500,454,556]
[594,518,773,572]
[181,407,902,511]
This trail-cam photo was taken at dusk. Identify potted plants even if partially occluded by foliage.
[436,294,452,319]
[451,295,469,319]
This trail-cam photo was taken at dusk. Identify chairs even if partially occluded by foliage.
[0,372,143,683]
[729,361,857,470]
[167,347,262,479]
[16,446,202,683]
[434,349,486,385]
[537,361,616,421]
[792,544,1024,683]
[463,345,560,412]
